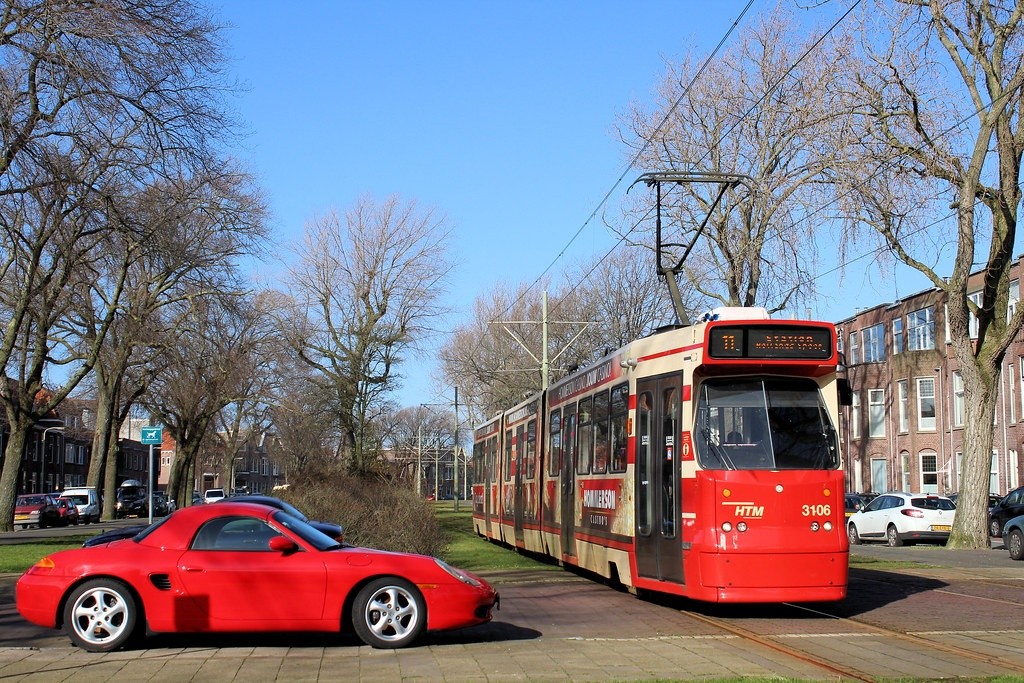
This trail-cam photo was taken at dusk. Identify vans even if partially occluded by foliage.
[204,488,226,505]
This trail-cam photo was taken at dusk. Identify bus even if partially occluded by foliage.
[470,304,852,610]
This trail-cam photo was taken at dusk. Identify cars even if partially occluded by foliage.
[845,491,891,525]
[989,485,1024,537]
[846,490,958,548]
[15,484,176,532]
[945,491,1007,536]
[82,495,345,560]
[1002,515,1024,560]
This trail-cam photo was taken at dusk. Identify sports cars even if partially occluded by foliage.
[14,502,502,653]
